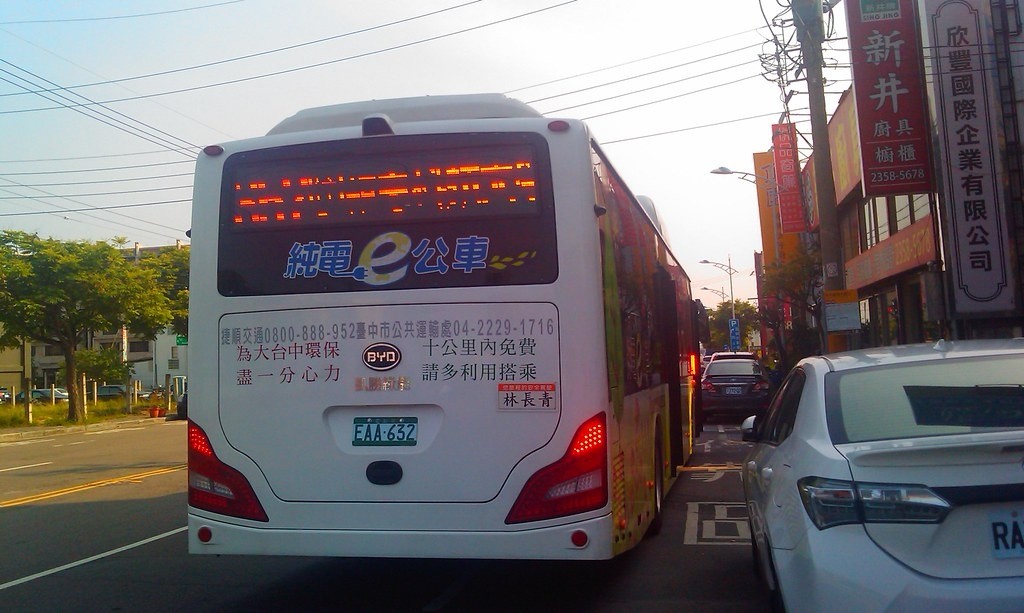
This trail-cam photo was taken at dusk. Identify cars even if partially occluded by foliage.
[15,389,57,404]
[90,384,140,399]
[743,338,1023,613]
[49,388,68,401]
[699,352,776,427]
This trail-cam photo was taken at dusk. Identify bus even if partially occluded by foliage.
[179,89,707,577]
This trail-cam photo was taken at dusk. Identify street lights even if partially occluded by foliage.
[698,257,737,353]
[709,163,789,367]
[699,284,729,354]
